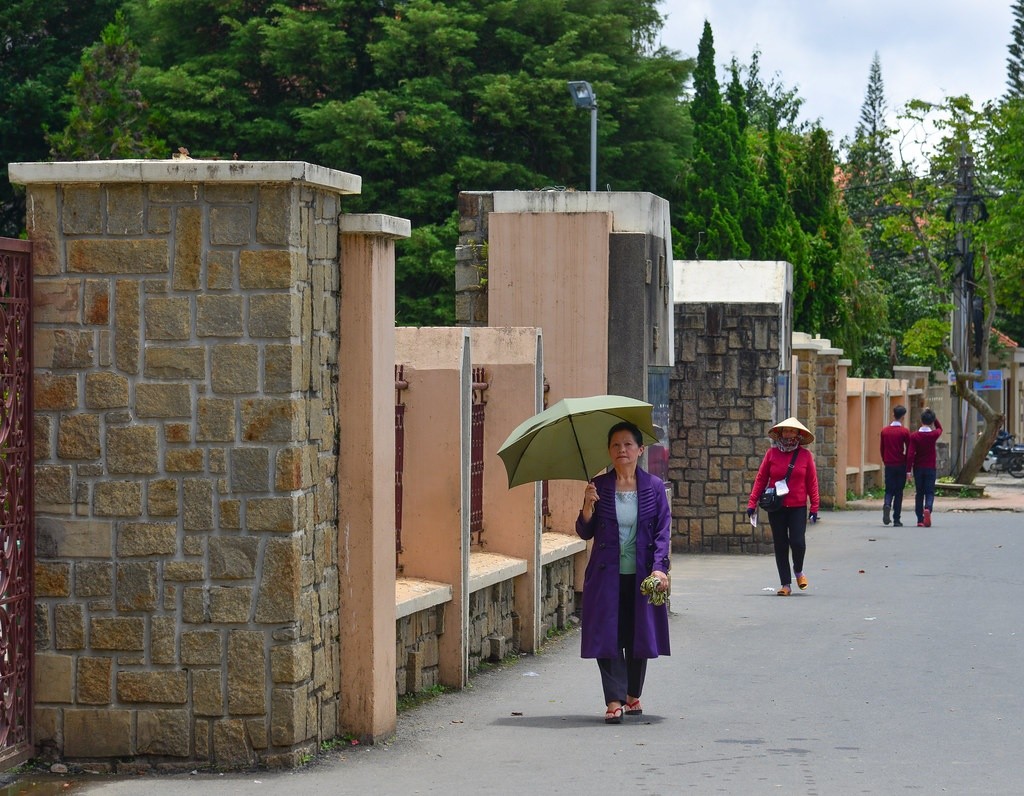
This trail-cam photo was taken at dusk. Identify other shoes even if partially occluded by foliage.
[924,508,932,526]
[917,521,924,526]
[893,519,904,526]
[882,504,891,525]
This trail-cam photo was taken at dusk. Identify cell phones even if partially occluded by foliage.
[810,515,814,526]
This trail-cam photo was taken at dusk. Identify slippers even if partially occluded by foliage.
[777,586,791,596]
[796,573,807,589]
[623,699,643,715]
[605,708,624,725]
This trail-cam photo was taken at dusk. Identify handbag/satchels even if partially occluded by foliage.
[758,489,781,512]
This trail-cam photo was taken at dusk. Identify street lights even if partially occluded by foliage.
[567,80,597,192]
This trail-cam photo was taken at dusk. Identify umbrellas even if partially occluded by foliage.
[496,395,661,504]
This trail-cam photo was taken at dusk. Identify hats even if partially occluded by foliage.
[767,417,815,445]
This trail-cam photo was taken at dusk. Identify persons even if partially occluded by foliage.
[576,421,672,724]
[747,417,820,595]
[907,407,944,527]
[880,405,915,527]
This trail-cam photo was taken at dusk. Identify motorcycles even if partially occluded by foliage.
[980,428,1024,479]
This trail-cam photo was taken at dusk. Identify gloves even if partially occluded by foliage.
[807,511,817,522]
[747,508,756,517]
